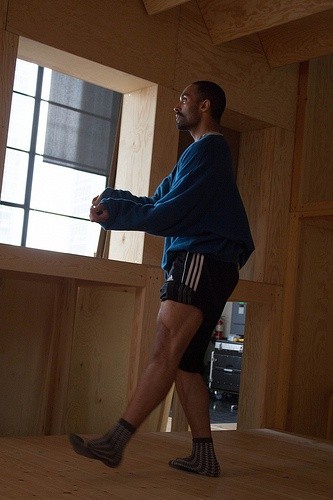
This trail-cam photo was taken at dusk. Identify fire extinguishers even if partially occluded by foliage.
[214,315,225,341]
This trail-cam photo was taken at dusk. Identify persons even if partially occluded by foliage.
[70,81,255,475]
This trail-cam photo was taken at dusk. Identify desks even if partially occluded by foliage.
[209,339,244,412]
